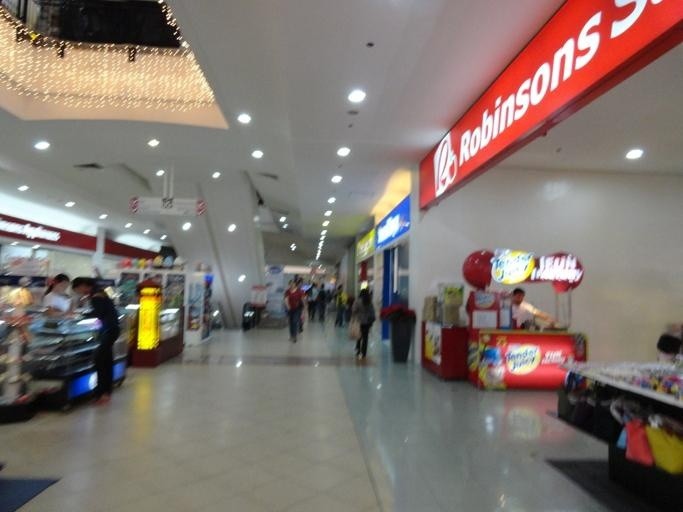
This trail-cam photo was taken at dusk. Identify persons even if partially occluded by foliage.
[42,273,74,317]
[510,288,557,328]
[284,274,375,361]
[656,333,680,360]
[71,277,120,406]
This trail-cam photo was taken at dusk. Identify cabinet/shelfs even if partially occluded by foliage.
[0,271,211,421]
